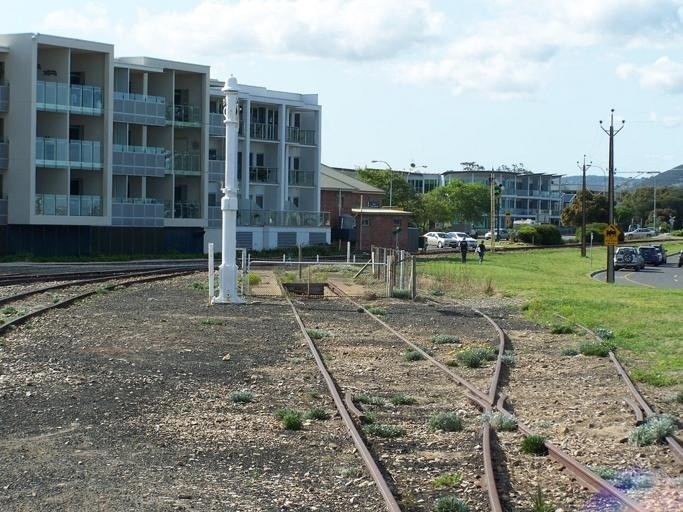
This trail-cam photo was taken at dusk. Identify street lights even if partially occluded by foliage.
[370,160,392,208]
[576,153,593,257]
[597,107,626,282]
[406,165,428,184]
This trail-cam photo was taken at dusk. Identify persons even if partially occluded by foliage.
[459,236,468,263]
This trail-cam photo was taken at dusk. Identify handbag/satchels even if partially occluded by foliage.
[474,244,481,253]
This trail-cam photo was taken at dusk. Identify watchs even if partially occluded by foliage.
[474,240,487,265]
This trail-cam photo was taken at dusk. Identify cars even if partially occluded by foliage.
[422,232,458,249]
[447,231,477,250]
[484,228,510,242]
[676,248,682,267]
[623,227,656,240]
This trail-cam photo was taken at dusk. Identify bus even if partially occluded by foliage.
[512,220,537,237]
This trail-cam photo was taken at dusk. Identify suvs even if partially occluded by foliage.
[614,244,669,272]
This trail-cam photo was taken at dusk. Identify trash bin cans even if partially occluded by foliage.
[418,236,427,251]
